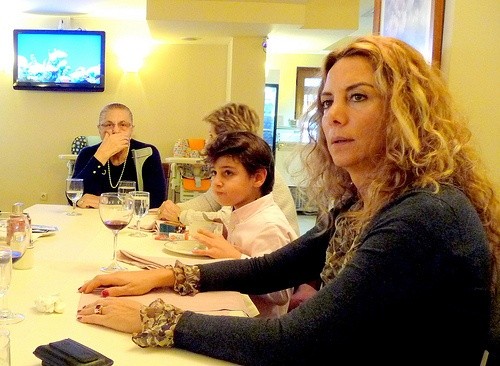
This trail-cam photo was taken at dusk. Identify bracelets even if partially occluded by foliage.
[178,212,183,226]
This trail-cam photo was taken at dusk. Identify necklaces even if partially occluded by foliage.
[108,140,130,188]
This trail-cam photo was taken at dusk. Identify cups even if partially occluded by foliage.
[0,212,31,265]
[118,181,136,201]
[0,328,12,366]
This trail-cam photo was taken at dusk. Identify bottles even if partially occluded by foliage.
[6,203,34,270]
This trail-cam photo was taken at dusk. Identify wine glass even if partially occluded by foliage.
[98,192,135,274]
[65,178,84,215]
[0,245,24,324]
[129,191,151,237]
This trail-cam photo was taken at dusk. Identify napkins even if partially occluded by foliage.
[116,247,179,269]
[130,220,164,234]
[78,291,261,318]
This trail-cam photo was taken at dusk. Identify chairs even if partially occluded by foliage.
[161,138,213,204]
[59,136,102,177]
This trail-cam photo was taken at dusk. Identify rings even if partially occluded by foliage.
[94,303,103,314]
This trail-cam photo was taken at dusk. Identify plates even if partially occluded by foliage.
[164,239,209,256]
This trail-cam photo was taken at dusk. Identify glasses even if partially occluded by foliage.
[99,121,131,130]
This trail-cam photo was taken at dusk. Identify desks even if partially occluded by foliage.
[0,205,248,366]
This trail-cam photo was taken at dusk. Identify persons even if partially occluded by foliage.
[158,101,301,240]
[190,132,298,319]
[77,36,500,366]
[66,103,167,211]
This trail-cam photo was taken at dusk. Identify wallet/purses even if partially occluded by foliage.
[33,338,115,366]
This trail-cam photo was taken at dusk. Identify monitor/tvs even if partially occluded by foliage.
[13,29,105,92]
